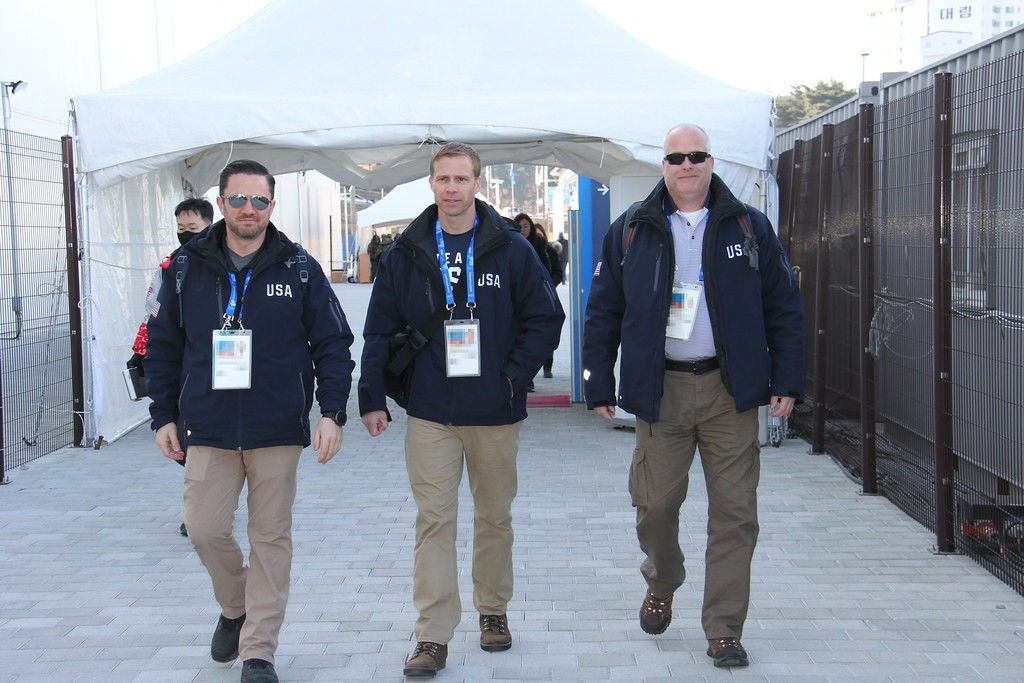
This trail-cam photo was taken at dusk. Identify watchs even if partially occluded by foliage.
[322,410,347,427]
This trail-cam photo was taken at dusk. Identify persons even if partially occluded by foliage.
[580,126,807,670]
[514,213,551,394]
[146,159,356,683]
[535,223,564,378]
[357,143,566,678]
[131,198,214,536]
[556,231,569,285]
[367,233,401,284]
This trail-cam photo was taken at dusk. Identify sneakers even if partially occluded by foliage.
[403,642,447,675]
[639,588,671,635]
[707,637,749,667]
[479,614,512,651]
[211,613,247,667]
[241,659,279,683]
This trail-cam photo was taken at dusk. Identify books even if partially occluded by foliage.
[122,368,147,400]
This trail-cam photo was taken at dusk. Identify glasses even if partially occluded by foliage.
[664,151,711,165]
[221,195,272,210]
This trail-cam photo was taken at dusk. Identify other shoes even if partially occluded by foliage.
[527,381,535,392]
[181,523,188,536]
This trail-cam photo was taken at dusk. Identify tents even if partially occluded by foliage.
[61,1,780,450]
[356,175,503,283]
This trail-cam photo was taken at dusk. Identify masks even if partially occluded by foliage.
[177,231,199,246]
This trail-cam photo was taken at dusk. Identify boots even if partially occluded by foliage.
[543,359,553,378]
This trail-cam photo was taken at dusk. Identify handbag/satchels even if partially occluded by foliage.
[382,326,428,405]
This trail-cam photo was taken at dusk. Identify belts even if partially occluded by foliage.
[665,357,719,375]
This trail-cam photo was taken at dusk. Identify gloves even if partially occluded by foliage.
[127,354,145,377]
